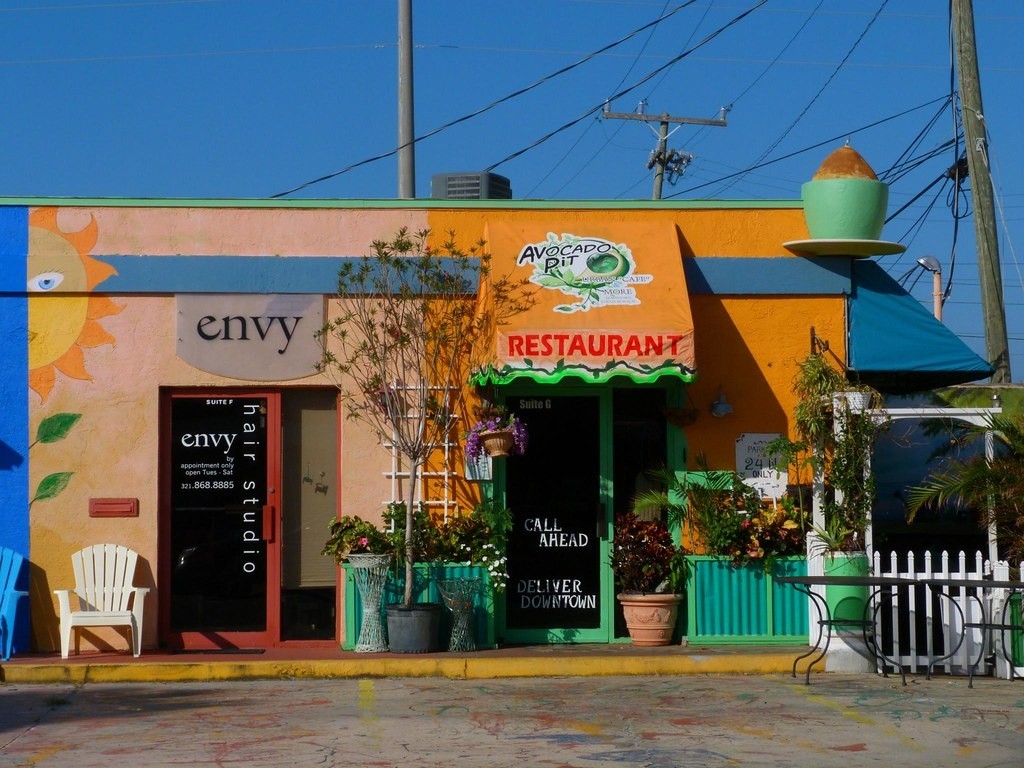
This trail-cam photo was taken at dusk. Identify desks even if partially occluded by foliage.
[923,580,1024,691]
[773,574,917,685]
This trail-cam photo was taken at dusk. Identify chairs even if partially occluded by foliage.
[0,546,30,661]
[54,544,150,659]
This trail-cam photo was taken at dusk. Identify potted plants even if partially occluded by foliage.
[762,353,872,630]
[900,383,1024,669]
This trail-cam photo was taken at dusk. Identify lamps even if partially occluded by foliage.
[711,395,731,418]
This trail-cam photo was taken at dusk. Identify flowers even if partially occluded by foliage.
[608,512,677,592]
[315,228,546,605]
[464,405,527,459]
[320,515,397,563]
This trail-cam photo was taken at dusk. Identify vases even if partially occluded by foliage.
[661,408,700,428]
[384,602,441,654]
[346,554,392,653]
[480,430,515,457]
[616,594,686,648]
[436,577,482,652]
